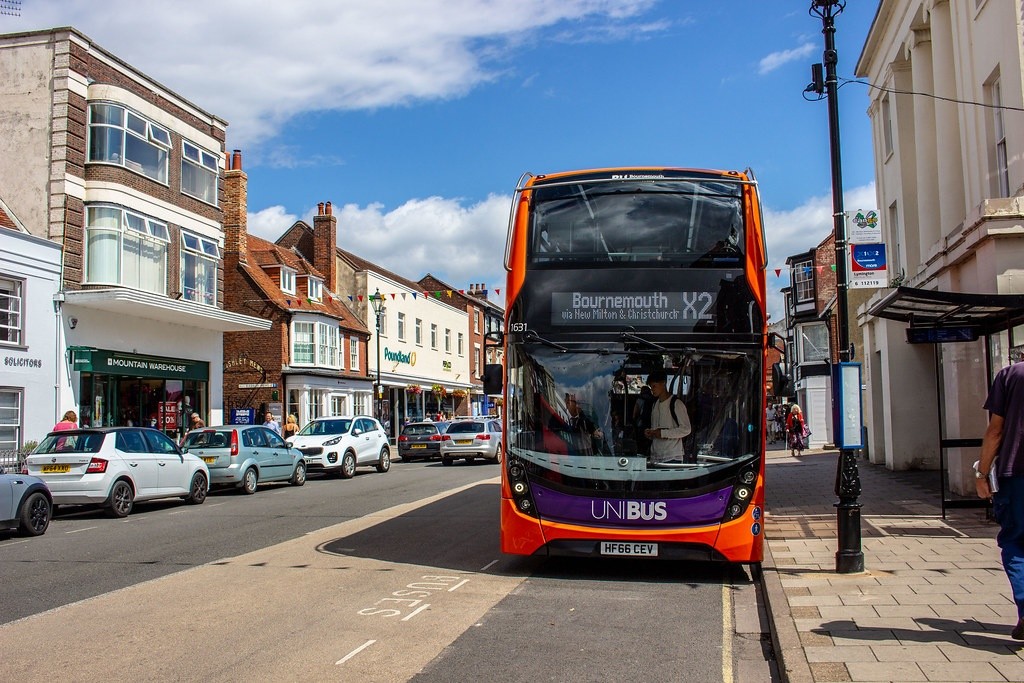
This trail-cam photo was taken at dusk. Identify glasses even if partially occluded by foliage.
[191,417,196,419]
[568,400,581,406]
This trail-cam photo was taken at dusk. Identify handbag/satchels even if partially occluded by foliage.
[64,423,76,451]
[802,424,811,439]
[972,456,999,493]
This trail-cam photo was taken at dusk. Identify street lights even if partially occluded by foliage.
[370,288,385,422]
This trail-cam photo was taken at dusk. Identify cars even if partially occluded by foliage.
[440,420,501,465]
[181,425,307,494]
[398,422,447,462]
[1,470,60,535]
[286,415,391,477]
[31,426,212,517]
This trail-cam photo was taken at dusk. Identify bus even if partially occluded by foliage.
[480,167,795,563]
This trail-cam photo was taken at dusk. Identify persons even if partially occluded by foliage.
[185,396,193,432]
[765,402,777,445]
[785,404,805,457]
[54,411,78,451]
[191,412,204,429]
[150,419,157,429]
[263,412,279,434]
[282,414,299,440]
[385,417,390,446]
[644,371,691,464]
[560,392,603,455]
[423,413,433,421]
[976,362,1024,639]
[436,412,446,422]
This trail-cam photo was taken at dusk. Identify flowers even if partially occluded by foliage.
[451,389,467,398]
[432,383,449,400]
[496,398,504,407]
[406,383,420,400]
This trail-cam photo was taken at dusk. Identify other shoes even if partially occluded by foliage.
[769,440,775,444]
[791,451,795,456]
[798,454,802,456]
[1012,616,1024,640]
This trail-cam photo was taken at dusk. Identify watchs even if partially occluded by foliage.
[976,472,989,479]
